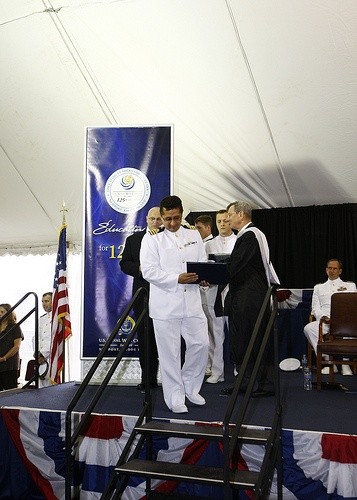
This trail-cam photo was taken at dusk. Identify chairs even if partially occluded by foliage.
[306,292,357,390]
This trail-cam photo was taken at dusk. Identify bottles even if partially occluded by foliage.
[303,355,308,366]
[304,366,312,390]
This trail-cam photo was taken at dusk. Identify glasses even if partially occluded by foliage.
[160,215,181,222]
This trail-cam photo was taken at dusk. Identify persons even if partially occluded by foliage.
[119,195,275,413]
[304,259,357,376]
[0,304,24,391]
[32,292,53,388]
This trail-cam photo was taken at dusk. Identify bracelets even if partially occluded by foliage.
[4,356,6,361]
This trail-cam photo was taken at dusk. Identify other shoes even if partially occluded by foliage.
[321,364,357,374]
[206,375,225,384]
[205,368,212,375]
[171,404,188,413]
[185,393,206,405]
[218,385,247,397]
[137,379,158,389]
[340,365,353,375]
[250,388,276,398]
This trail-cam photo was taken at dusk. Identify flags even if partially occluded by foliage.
[48,224,72,385]
